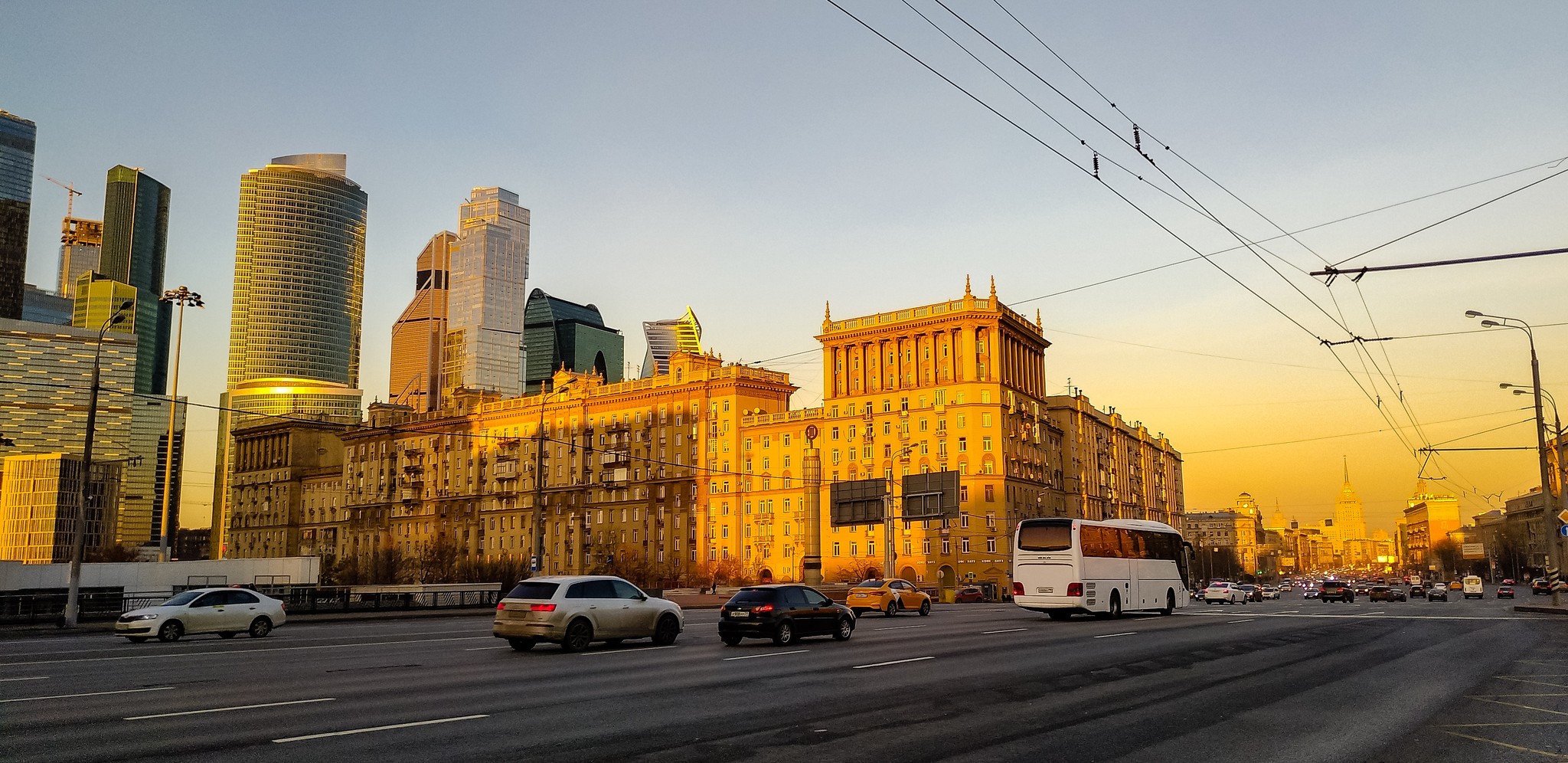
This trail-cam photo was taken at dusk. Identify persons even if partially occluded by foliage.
[1001,593,1006,602]
[711,582,716,595]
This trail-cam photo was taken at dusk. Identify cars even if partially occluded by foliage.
[1278,568,1409,602]
[115,587,286,644]
[493,575,684,653]
[1189,579,1279,605]
[1502,579,1515,586]
[846,577,931,618]
[1496,587,1514,599]
[1530,579,1567,595]
[1409,583,1448,602]
[950,588,983,603]
[718,584,856,647]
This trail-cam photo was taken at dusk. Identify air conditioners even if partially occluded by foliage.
[737,479,743,485]
[613,483,617,486]
[859,471,866,477]
[978,469,985,475]
[687,434,690,438]
[895,504,901,509]
[1006,397,1053,485]
[990,526,998,532]
[649,476,654,479]
[950,399,957,405]
[846,438,852,442]
[935,431,946,435]
[602,483,606,487]
[825,409,864,417]
[930,401,935,408]
[718,432,726,437]
[576,478,579,482]
[704,532,709,538]
[895,424,900,428]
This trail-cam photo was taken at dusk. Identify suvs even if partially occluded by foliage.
[1423,581,1432,589]
[1320,580,1354,603]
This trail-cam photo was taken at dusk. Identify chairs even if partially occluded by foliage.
[1080,540,1166,565]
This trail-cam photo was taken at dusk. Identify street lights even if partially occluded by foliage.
[157,287,205,562]
[890,442,919,577]
[1499,383,1568,583]
[1242,542,1253,584]
[1253,544,1263,582]
[1464,310,1561,607]
[65,300,133,628]
[533,377,579,577]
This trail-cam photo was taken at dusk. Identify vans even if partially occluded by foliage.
[1411,576,1420,585]
[1463,575,1483,600]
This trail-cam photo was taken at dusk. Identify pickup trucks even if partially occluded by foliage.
[1449,582,1462,591]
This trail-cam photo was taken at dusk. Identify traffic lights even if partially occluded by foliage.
[1256,570,1262,575]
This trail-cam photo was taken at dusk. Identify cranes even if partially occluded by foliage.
[37,171,82,244]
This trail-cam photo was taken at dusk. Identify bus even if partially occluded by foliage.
[1012,518,1196,620]
